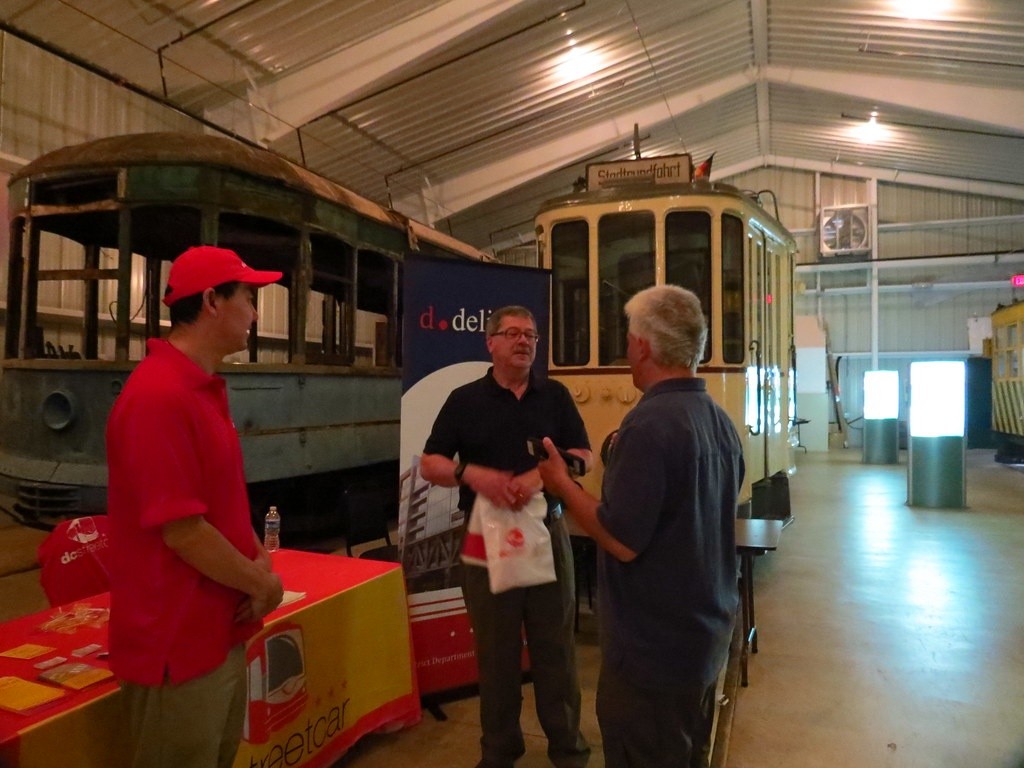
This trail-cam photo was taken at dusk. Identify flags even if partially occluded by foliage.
[692,155,714,182]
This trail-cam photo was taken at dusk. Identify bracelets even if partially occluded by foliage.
[454,463,466,483]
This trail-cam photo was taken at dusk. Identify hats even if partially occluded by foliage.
[162,246,283,307]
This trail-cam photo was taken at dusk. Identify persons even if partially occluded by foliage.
[107,246,285,768]
[417,304,593,768]
[540,283,744,767]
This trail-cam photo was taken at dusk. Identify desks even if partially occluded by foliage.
[735,519,785,687]
[0,547,425,768]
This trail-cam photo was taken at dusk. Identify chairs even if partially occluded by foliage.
[340,488,399,562]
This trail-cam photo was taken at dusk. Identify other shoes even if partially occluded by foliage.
[474,748,526,768]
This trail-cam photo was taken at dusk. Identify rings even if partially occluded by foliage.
[520,493,523,497]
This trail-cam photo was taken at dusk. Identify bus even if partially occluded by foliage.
[528,122,800,572]
[0,132,499,532]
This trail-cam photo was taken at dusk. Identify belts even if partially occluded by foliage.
[544,504,561,526]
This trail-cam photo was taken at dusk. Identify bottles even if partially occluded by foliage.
[264,506,280,552]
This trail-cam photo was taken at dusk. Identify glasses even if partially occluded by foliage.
[491,327,539,341]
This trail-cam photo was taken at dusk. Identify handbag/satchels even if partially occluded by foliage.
[480,491,558,595]
[460,492,487,568]
